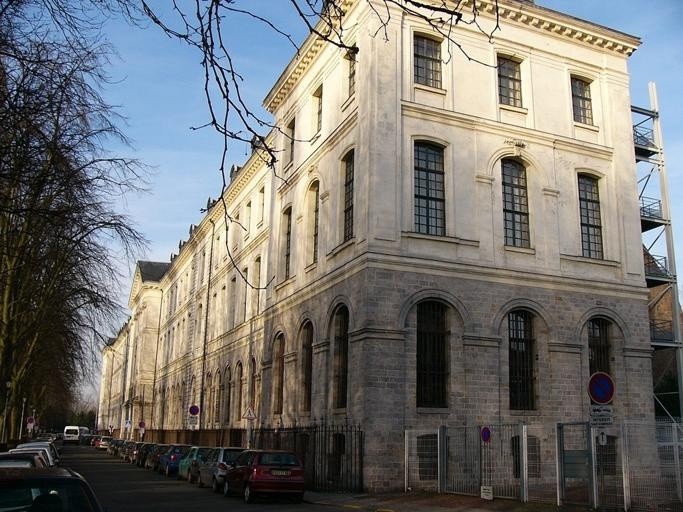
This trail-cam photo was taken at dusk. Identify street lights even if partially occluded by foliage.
[3,382,37,446]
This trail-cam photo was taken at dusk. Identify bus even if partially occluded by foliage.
[79,427,89,434]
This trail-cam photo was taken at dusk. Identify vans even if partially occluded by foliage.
[62,425,81,446]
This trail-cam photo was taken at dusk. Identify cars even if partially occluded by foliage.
[105,434,308,506]
[0,433,112,512]
